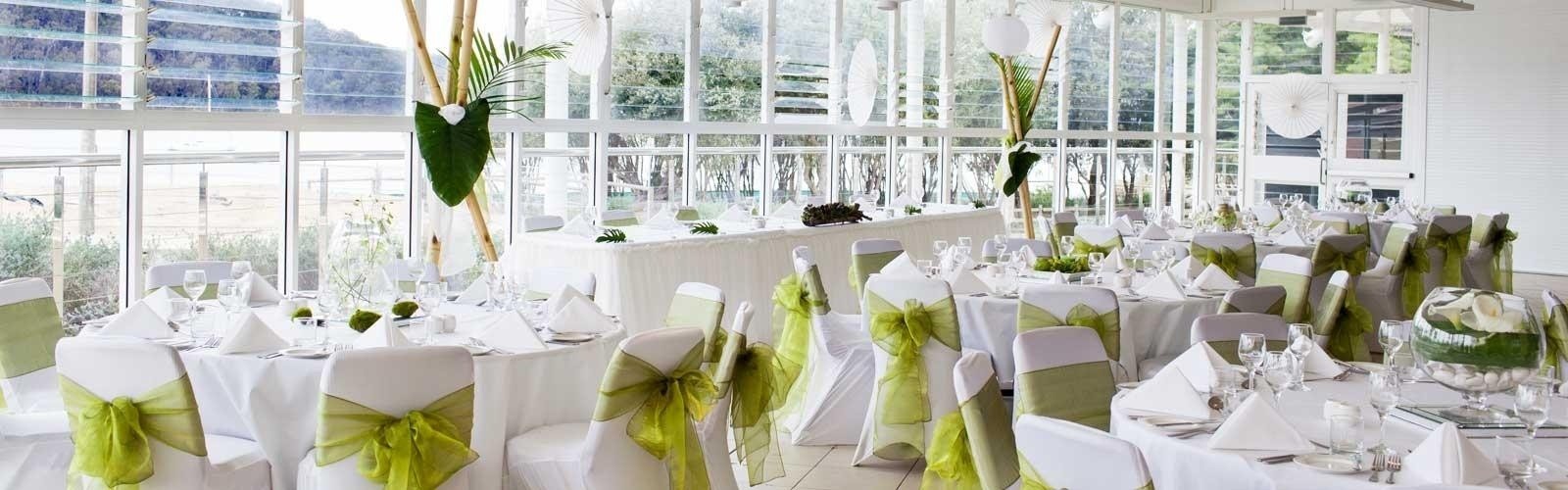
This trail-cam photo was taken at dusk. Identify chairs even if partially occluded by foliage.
[515,262,599,307]
[659,279,728,370]
[769,189,1511,361]
[1010,411,1156,490]
[667,205,700,222]
[520,211,564,233]
[293,344,477,490]
[948,348,1024,488]
[379,254,448,292]
[596,207,639,228]
[697,300,759,490]
[1010,324,1117,431]
[145,258,239,303]
[0,272,71,490]
[788,242,870,447]
[853,273,963,468]
[51,333,276,490]
[501,326,705,490]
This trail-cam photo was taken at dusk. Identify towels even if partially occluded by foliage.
[540,282,579,311]
[222,312,286,354]
[545,298,617,336]
[239,271,284,307]
[92,301,176,342]
[344,314,414,347]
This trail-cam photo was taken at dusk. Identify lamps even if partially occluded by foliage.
[983,0,1028,56]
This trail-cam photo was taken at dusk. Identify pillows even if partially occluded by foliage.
[476,309,547,351]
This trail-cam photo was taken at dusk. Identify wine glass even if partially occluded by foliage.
[180,248,548,359]
[1133,188,1435,243]
[1237,318,1553,490]
[911,234,1178,300]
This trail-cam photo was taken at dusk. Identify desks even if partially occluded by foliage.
[475,202,1007,349]
[932,270,1250,396]
[1110,359,1567,490]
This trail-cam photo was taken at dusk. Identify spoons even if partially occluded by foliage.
[1208,397,1224,416]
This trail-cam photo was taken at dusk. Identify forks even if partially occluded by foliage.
[1166,425,1222,441]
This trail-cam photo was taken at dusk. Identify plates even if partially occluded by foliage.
[1136,414,1202,433]
[553,330,595,342]
